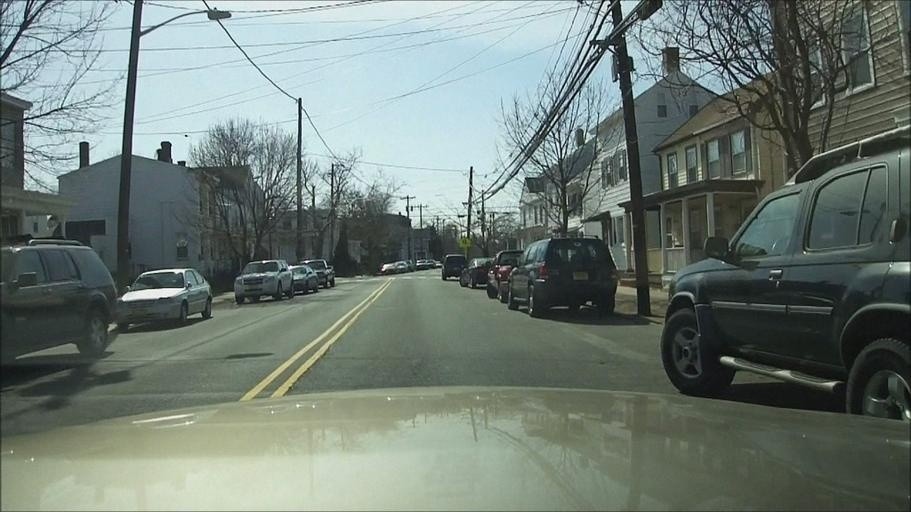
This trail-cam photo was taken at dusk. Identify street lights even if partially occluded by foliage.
[112,2,234,294]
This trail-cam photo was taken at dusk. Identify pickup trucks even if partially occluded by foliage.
[298,258,336,289]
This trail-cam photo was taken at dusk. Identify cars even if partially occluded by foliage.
[0,387,910,512]
[381,258,443,275]
[459,257,496,289]
[289,265,319,294]
[115,268,213,330]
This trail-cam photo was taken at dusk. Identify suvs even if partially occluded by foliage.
[487,249,525,304]
[0,238,122,356]
[661,126,910,422]
[442,254,467,281]
[232,258,295,304]
[506,234,621,317]
